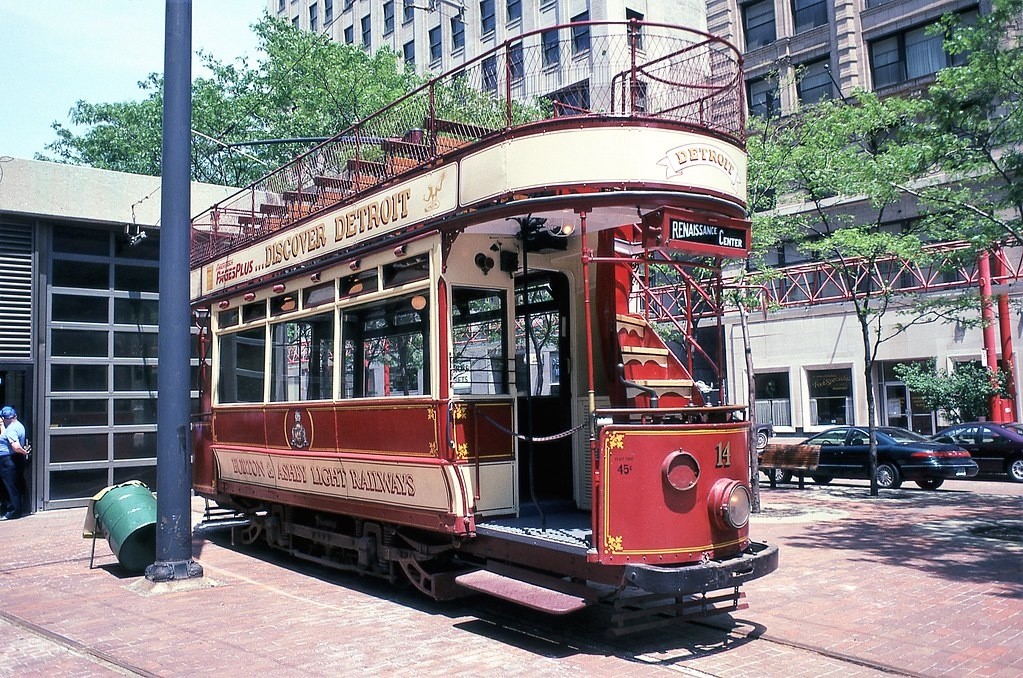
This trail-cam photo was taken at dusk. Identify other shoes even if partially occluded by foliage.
[0,516,9,521]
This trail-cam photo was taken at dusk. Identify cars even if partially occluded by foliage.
[930,422,1023,482]
[733,416,776,449]
[758,426,980,489]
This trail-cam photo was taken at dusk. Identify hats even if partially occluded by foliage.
[0,406,15,419]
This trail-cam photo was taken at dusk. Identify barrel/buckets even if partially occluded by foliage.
[93,484,157,575]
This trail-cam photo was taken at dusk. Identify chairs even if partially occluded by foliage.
[853,439,863,445]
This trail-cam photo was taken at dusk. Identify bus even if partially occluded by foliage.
[191,22,779,638]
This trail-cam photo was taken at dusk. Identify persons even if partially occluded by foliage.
[0,406,29,521]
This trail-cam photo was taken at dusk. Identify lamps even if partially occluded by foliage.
[122,224,151,246]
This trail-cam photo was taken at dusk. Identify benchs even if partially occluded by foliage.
[758,444,821,490]
[238,117,495,243]
